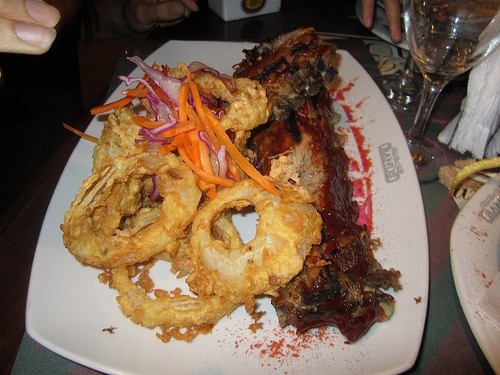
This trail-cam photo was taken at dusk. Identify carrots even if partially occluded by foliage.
[63,77,277,198]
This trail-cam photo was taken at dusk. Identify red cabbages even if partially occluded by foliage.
[120,56,236,200]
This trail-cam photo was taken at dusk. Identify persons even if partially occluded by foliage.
[363,0,403,42]
[0,0,199,199]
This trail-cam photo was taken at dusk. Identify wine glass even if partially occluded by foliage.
[387,0,500,184]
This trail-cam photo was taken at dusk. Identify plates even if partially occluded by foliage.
[449,172,500,375]
[24,40,430,375]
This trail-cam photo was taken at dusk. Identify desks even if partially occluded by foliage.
[0,0,500,375]
